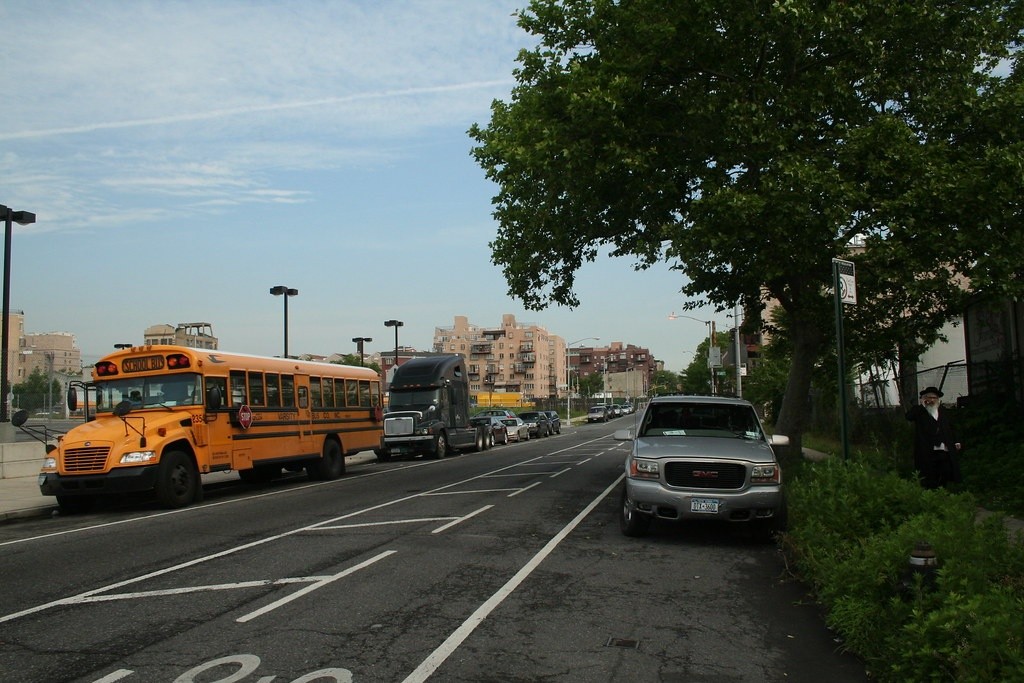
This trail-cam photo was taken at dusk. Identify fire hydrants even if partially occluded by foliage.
[903,539,939,588]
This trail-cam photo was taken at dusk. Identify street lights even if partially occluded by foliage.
[668,312,714,396]
[352,337,372,367]
[568,337,600,425]
[384,320,404,366]
[270,286,298,358]
[0,204,36,422]
[626,364,654,403]
[604,353,626,403]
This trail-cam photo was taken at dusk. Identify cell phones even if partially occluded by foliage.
[925,400,929,404]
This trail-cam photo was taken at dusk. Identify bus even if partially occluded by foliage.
[12,344,385,515]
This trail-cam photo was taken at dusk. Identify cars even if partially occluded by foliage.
[614,396,789,537]
[588,402,634,423]
[470,410,561,447]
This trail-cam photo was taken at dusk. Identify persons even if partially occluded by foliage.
[905,387,962,490]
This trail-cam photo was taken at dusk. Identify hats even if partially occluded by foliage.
[920,386,944,397]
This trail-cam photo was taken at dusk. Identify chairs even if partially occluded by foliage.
[661,410,678,428]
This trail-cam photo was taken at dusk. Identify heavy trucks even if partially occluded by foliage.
[374,356,490,462]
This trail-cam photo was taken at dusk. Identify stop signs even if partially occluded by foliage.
[238,405,253,430]
